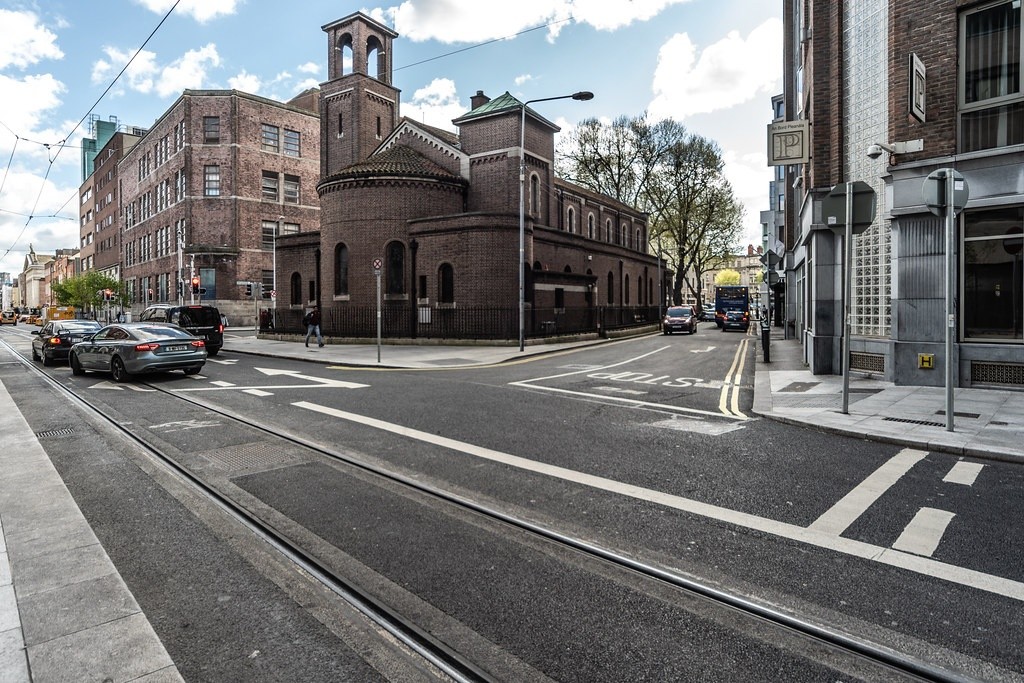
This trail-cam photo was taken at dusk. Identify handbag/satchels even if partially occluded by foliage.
[224,319,227,327]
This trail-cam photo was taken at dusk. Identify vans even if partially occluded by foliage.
[138,303,224,357]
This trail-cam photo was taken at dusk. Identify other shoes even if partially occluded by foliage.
[319,343,324,347]
[305,342,308,347]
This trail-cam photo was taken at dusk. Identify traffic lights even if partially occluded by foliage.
[179,283,182,296]
[102,291,104,300]
[200,288,206,295]
[106,289,110,300]
[149,290,152,300]
[245,284,251,295]
[192,278,199,294]
[111,296,114,301]
[262,285,266,295]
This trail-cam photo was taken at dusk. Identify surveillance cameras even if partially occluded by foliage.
[867,145,883,158]
[792,176,803,189]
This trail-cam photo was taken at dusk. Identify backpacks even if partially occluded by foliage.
[303,312,314,327]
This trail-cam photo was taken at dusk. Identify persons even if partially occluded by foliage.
[220,313,228,330]
[762,304,766,318]
[116,312,121,323]
[259,308,275,334]
[306,305,324,347]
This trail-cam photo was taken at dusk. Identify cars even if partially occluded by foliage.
[699,305,715,322]
[662,305,698,335]
[0,309,17,326]
[68,322,208,382]
[721,311,750,332]
[30,319,103,367]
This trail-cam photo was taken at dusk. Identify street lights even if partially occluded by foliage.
[519,91,594,353]
[272,215,285,330]
[658,246,686,331]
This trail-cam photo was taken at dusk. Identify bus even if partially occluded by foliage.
[715,286,748,328]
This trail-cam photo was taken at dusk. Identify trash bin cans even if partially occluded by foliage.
[124,311,132,324]
